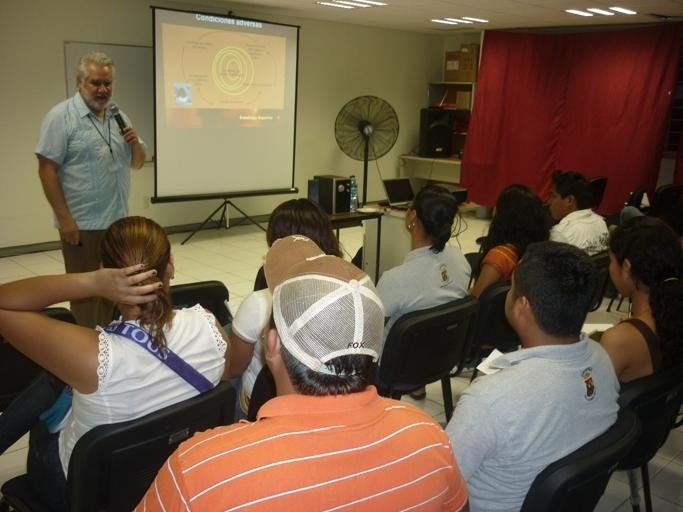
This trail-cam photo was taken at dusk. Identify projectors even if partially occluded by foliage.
[432,182,467,203]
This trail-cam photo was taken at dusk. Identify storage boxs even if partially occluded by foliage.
[444,43,481,111]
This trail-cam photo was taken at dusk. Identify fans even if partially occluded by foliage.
[335,95,400,209]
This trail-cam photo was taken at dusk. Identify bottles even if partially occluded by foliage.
[349,175,358,213]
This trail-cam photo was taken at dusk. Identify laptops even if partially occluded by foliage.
[382,178,416,210]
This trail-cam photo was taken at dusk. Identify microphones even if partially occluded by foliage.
[109,102,125,135]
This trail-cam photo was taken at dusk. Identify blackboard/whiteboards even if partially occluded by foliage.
[63,40,155,163]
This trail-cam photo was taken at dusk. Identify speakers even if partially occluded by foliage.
[419,106,453,159]
[313,175,351,214]
[308,180,319,205]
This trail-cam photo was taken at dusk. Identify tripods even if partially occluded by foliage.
[180,199,268,246]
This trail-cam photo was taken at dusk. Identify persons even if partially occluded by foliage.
[34,51,147,330]
[223,198,344,424]
[471,184,553,301]
[547,168,611,256]
[596,216,683,387]
[376,185,472,399]
[130,236,469,512]
[442,241,620,512]
[0,216,232,512]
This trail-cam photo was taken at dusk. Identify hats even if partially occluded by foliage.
[264,234,385,376]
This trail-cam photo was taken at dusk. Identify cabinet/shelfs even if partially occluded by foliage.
[399,81,475,198]
